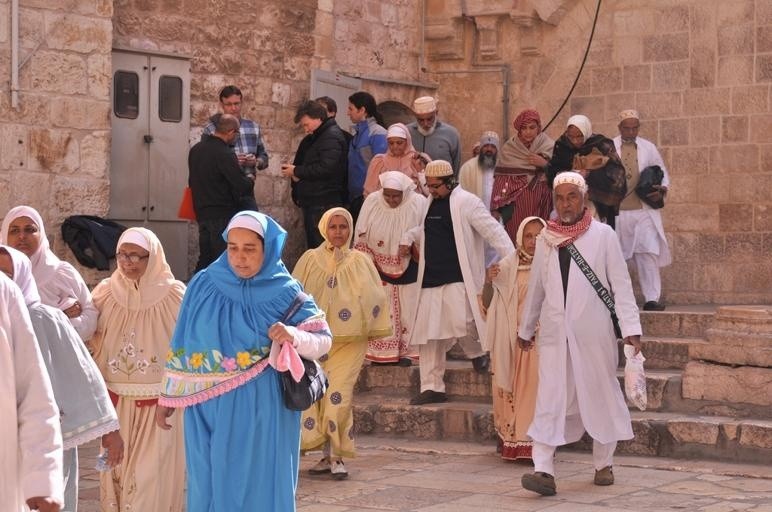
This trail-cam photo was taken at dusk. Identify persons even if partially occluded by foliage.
[0,244,125,510]
[288,206,394,482]
[61,225,190,510]
[1,204,99,346]
[153,211,332,510]
[480,215,547,465]
[517,170,645,496]
[0,270,65,510]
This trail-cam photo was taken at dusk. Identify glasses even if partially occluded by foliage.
[117,252,148,263]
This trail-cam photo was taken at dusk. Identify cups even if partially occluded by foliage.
[279,155,292,178]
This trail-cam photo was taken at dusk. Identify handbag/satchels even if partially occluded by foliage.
[276,293,328,409]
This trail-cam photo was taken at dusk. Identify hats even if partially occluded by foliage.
[618,108,640,125]
[424,159,454,177]
[412,96,436,114]
[552,171,587,190]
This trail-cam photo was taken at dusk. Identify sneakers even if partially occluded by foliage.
[595,466,614,486]
[521,472,556,497]
[328,461,349,479]
[643,301,665,311]
[473,354,490,373]
[308,459,330,475]
[410,390,448,406]
